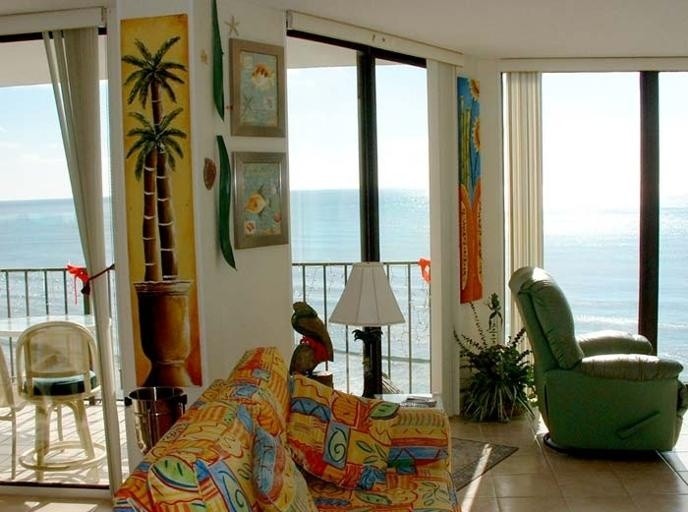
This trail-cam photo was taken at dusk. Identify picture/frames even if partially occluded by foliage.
[228,38,286,137]
[231,151,289,250]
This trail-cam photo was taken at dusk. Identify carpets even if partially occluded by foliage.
[451,437,519,492]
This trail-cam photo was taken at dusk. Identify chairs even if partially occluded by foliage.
[0,315,112,470]
[0,349,63,480]
[16,321,102,482]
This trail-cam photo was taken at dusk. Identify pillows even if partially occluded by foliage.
[253,425,316,512]
[286,374,401,495]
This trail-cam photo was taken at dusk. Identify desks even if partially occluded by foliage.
[374,394,443,409]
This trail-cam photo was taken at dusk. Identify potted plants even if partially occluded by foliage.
[121,34,195,387]
[452,293,536,423]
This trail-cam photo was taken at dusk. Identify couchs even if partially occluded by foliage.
[111,346,461,512]
[508,266,688,455]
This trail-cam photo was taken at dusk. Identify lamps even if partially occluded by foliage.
[328,262,405,397]
